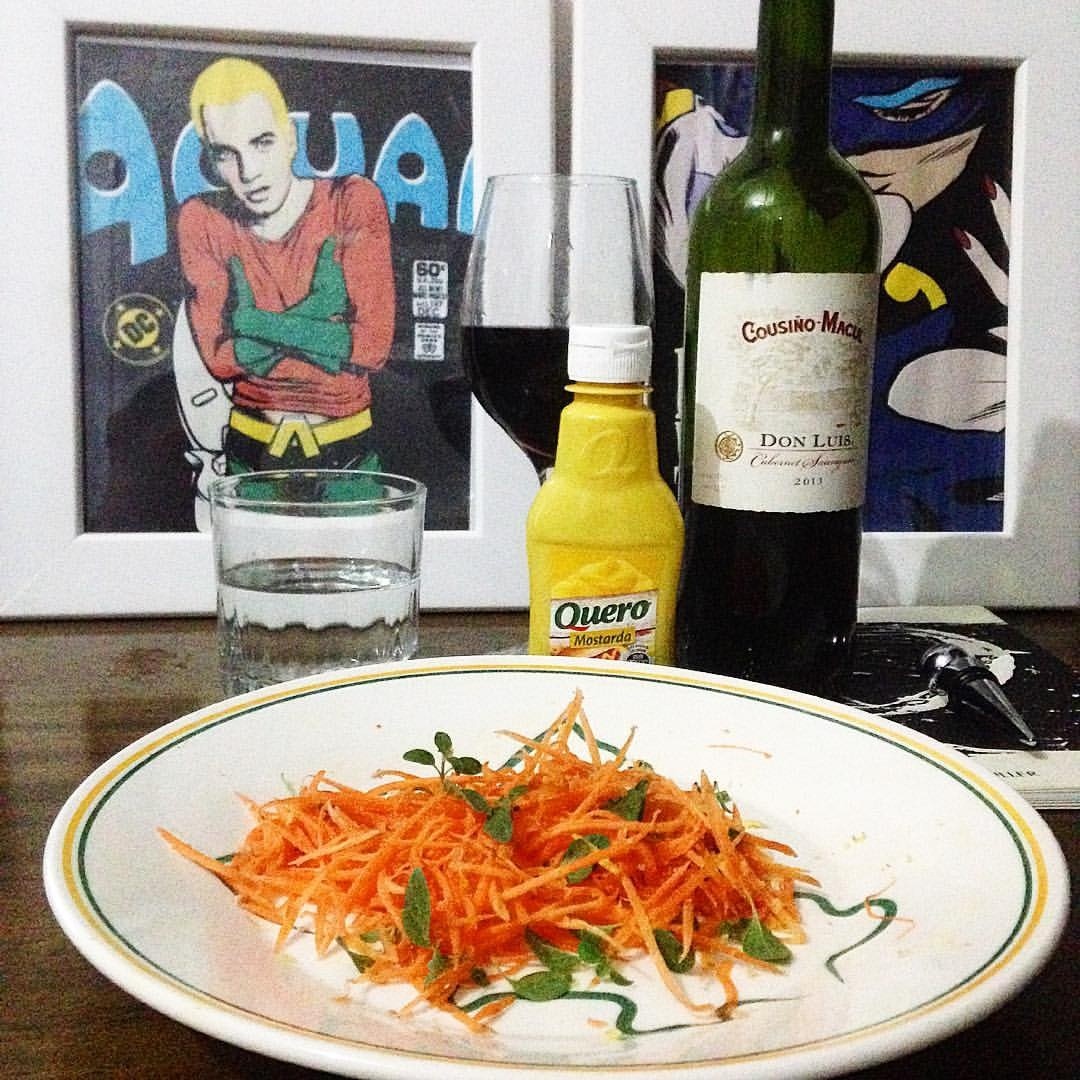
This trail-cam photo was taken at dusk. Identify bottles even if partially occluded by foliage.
[678,0,883,691]
[530,323,686,668]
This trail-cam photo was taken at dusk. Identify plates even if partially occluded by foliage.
[43,651,1071,1080]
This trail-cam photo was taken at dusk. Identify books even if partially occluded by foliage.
[851,605,1080,809]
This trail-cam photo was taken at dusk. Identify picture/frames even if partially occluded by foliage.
[0,0,559,622]
[566,0,1079,609]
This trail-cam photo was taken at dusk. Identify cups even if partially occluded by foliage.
[208,469,426,698]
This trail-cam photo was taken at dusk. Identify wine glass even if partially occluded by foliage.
[461,173,654,655]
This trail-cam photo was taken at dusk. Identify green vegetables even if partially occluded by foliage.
[339,731,790,1002]
